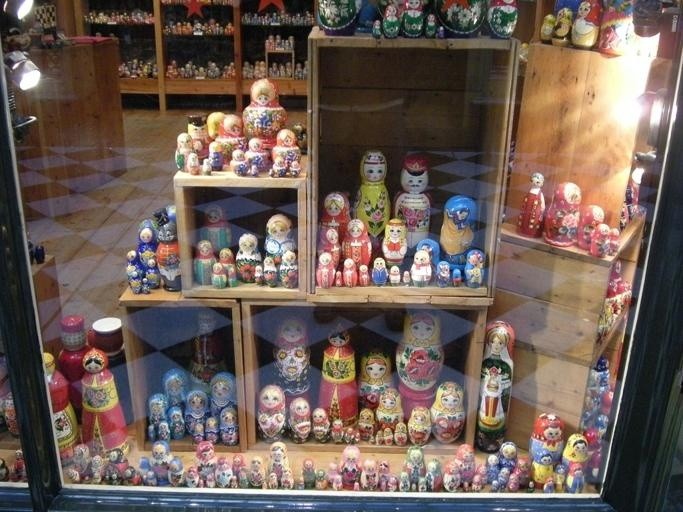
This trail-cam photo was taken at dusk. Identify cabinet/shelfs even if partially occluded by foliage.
[117,40,520,452]
[16,39,128,220]
[73,0,317,113]
[487,44,673,451]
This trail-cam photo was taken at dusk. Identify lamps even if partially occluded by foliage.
[0,0,55,44]
[2,50,41,140]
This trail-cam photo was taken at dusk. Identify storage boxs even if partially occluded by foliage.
[174,157,309,297]
